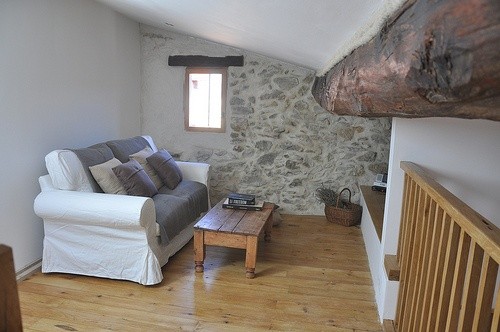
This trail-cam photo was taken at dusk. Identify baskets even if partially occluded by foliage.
[325,188,362,227]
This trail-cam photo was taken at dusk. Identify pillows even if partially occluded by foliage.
[88,157,128,195]
[111,158,158,198]
[146,147,184,191]
[128,145,164,190]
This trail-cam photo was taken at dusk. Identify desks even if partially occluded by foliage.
[193,196,276,278]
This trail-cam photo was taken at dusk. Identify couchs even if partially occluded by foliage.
[33,136,213,285]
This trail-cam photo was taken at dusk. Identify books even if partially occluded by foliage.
[222,194,265,211]
